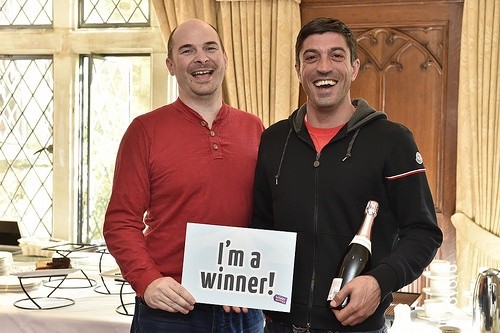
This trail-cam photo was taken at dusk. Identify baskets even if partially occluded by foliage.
[18,237,55,258]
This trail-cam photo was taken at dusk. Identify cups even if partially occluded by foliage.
[424,300,451,318]
[429,260,457,276]
[430,297,457,307]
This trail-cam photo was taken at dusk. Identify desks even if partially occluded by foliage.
[0,238,473,333]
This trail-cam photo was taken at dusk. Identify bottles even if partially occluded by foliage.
[329,200,381,309]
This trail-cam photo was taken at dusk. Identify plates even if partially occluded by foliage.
[98,268,122,277]
[423,288,457,296]
[9,269,78,276]
[423,272,457,279]
[429,279,458,292]
[417,312,454,323]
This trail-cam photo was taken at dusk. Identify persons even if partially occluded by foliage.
[223,17,443,333]
[103,19,265,333]
